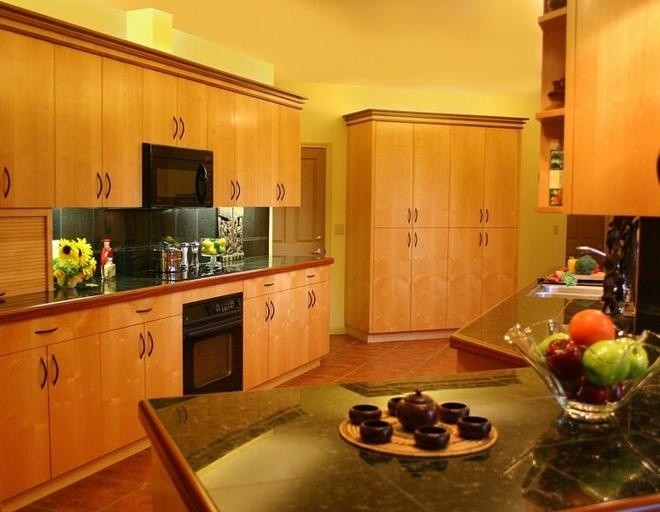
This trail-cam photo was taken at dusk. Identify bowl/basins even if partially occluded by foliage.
[348,404,382,425]
[456,415,491,439]
[388,397,405,415]
[439,402,470,424]
[360,419,393,443]
[414,424,450,450]
[504,318,660,423]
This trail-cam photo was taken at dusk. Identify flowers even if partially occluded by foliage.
[52,236,98,280]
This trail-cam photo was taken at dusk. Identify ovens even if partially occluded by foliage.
[183,292,243,395]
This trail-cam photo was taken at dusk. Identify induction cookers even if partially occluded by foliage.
[126,264,240,281]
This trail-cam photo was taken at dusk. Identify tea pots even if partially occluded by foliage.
[395,387,440,431]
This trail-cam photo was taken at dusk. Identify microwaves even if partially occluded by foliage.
[142,142,213,208]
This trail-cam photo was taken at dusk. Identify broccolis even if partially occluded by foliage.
[574,255,599,275]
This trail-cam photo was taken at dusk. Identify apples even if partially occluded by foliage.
[200,237,227,255]
[582,340,631,386]
[549,338,582,377]
[616,337,649,378]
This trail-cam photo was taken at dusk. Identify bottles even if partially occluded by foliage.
[100,238,113,279]
[180,265,188,280]
[104,257,116,279]
[180,242,190,268]
[190,241,200,267]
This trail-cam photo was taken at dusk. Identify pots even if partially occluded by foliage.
[151,241,182,273]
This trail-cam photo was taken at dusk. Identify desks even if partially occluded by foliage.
[137,367,660,511]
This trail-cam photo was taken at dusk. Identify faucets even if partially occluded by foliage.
[575,245,626,297]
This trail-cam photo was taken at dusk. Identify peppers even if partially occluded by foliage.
[567,258,577,274]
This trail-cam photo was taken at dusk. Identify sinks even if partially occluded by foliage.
[531,283,604,298]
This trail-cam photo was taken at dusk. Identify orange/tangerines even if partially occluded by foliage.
[568,308,614,347]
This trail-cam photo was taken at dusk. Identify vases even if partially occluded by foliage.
[56,270,81,290]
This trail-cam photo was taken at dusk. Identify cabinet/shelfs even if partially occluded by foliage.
[0,30,56,208]
[258,93,303,209]
[449,228,520,330]
[345,227,449,334]
[534,0,659,218]
[450,114,519,227]
[347,113,450,227]
[290,263,330,374]
[145,62,209,151]
[209,83,258,208]
[57,42,145,210]
[102,289,183,458]
[244,269,290,392]
[1,306,101,502]
